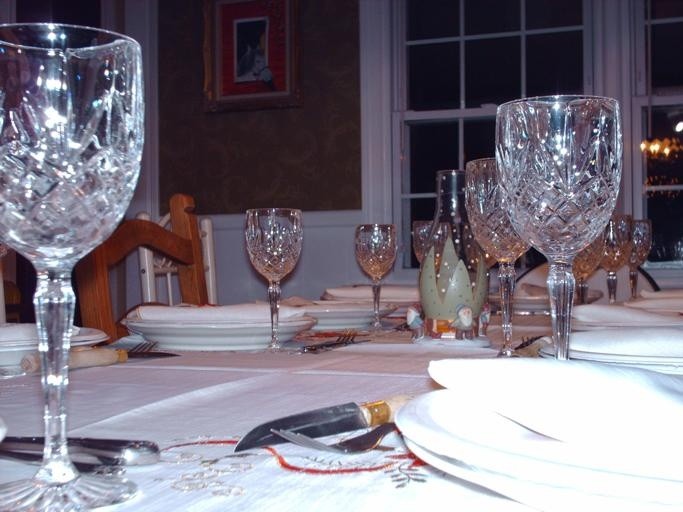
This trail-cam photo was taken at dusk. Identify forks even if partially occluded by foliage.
[268,423,395,455]
[130,341,156,354]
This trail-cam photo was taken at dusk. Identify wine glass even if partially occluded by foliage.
[0,20,146,512]
[494,93,626,360]
[573,229,606,306]
[600,213,632,305]
[354,224,399,336]
[464,158,533,357]
[246,205,304,358]
[625,219,654,300]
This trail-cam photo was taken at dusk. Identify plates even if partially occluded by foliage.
[325,285,423,318]
[486,289,603,314]
[395,287,683,512]
[256,297,398,330]
[122,318,317,351]
[0,322,111,371]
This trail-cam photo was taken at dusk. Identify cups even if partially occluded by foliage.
[411,221,452,270]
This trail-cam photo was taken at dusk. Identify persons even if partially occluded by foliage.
[404,302,426,343]
[448,303,477,340]
[237,48,265,83]
[478,302,491,336]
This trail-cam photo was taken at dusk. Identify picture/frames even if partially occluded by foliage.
[198,0,301,113]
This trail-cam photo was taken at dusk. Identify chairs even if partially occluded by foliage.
[515,255,661,298]
[69,191,217,345]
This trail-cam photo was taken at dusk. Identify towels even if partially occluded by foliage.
[425,355,683,483]
[624,298,683,313]
[569,301,683,326]
[1,318,80,343]
[322,285,422,302]
[554,323,683,358]
[126,297,306,321]
[639,288,683,298]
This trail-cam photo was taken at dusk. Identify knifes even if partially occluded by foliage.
[233,395,412,454]
[18,348,182,375]
